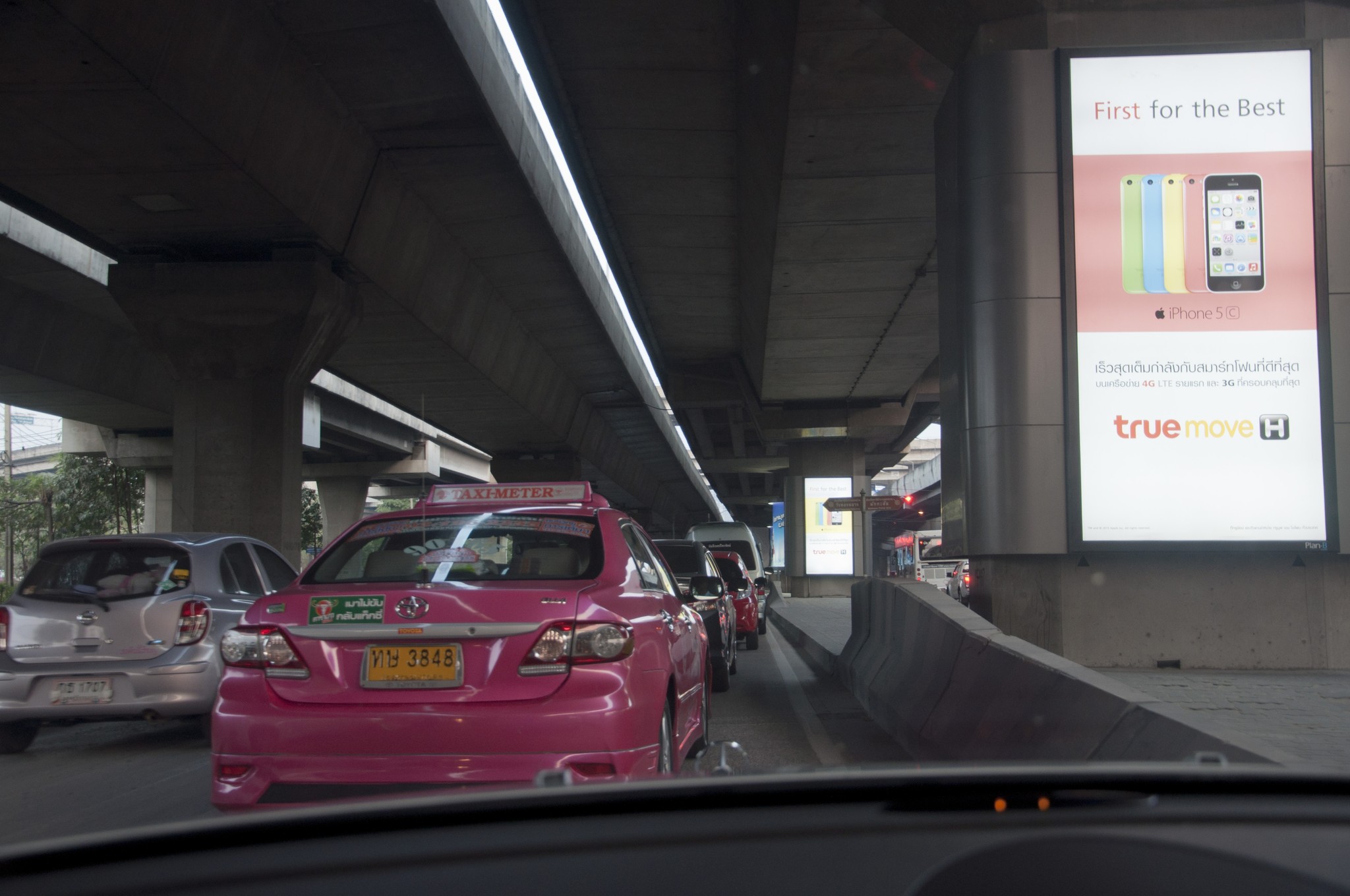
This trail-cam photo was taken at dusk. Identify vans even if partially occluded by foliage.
[684,520,774,634]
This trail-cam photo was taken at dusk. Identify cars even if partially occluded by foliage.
[0,530,304,755]
[211,479,773,808]
[945,560,971,607]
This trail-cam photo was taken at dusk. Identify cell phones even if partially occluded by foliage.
[1202,172,1266,295]
[1120,173,1150,296]
[1161,173,1194,294]
[1140,175,1167,294]
[1183,171,1213,293]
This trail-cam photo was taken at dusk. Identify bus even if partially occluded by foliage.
[891,529,968,588]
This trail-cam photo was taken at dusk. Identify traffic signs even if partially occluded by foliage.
[822,496,905,511]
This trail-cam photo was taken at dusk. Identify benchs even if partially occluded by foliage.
[364,546,581,581]
